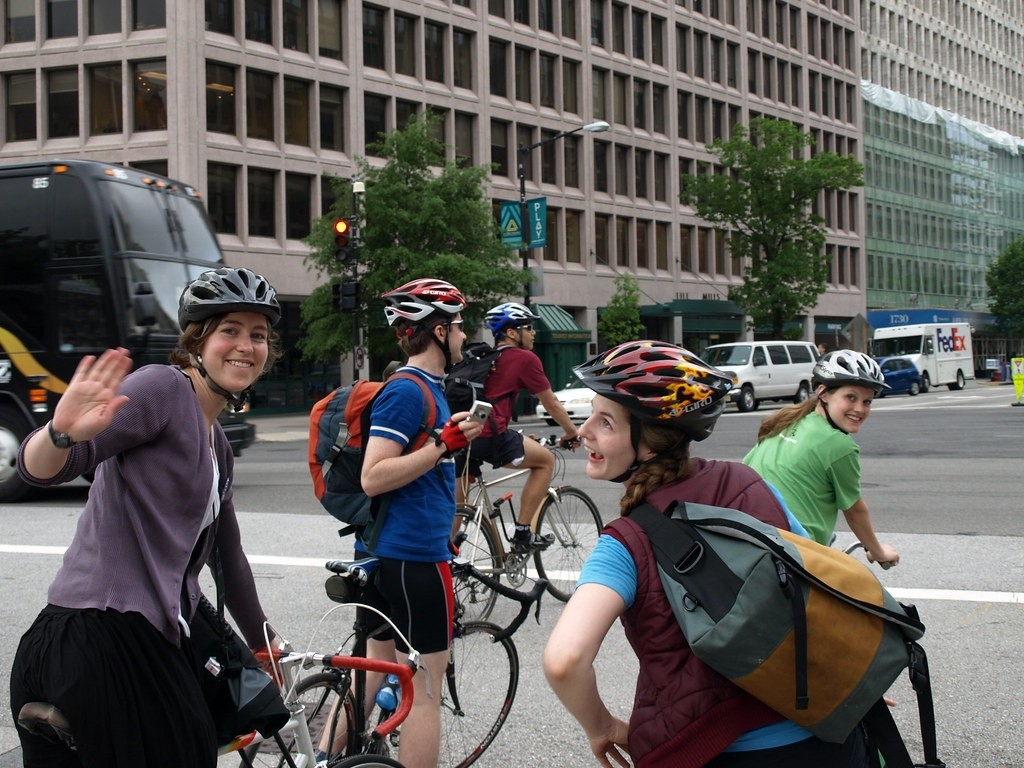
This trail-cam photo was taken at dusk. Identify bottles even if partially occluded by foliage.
[375,673,402,711]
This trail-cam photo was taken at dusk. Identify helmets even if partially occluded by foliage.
[380,278,467,327]
[573,340,733,442]
[811,350,892,397]
[179,268,281,327]
[485,302,541,333]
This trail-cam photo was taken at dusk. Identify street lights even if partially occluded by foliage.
[516,121,613,307]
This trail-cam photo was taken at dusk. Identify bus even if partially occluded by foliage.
[0,159,260,502]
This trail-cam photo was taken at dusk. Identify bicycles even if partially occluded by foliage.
[211,505,522,768]
[10,601,417,768]
[448,434,609,637]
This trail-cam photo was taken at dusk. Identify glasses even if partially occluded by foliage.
[440,320,464,332]
[506,324,533,334]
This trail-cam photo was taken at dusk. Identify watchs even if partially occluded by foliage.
[49,419,78,449]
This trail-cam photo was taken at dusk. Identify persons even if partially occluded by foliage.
[315,279,482,768]
[8,266,278,768]
[452,302,581,553]
[744,349,900,566]
[818,343,829,355]
[544,340,896,768]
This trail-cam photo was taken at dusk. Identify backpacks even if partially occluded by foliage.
[309,361,437,529]
[444,342,518,421]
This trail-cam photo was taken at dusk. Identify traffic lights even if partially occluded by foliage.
[332,218,350,264]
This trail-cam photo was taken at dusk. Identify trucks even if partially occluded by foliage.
[868,323,975,393]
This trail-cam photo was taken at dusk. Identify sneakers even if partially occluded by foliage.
[511,533,554,553]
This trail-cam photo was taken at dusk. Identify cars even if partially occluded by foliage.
[537,378,596,427]
[871,357,925,399]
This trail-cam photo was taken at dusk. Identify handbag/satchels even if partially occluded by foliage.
[623,499,924,744]
[180,592,290,751]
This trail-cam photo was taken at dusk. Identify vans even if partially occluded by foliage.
[697,340,824,416]
